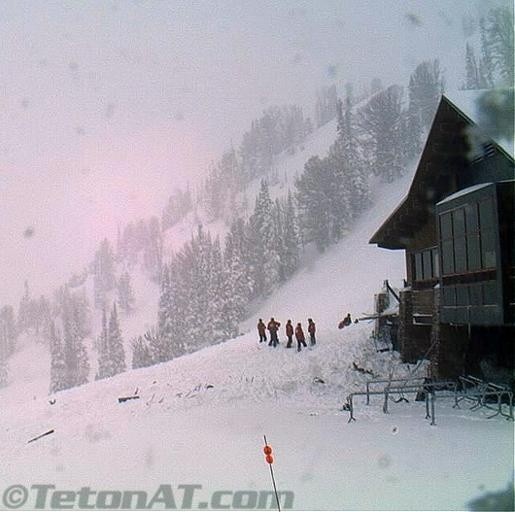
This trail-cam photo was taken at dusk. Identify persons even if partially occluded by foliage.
[338,313,352,330]
[266,317,280,346]
[307,318,316,346]
[257,318,266,343]
[295,322,307,352]
[285,319,294,348]
